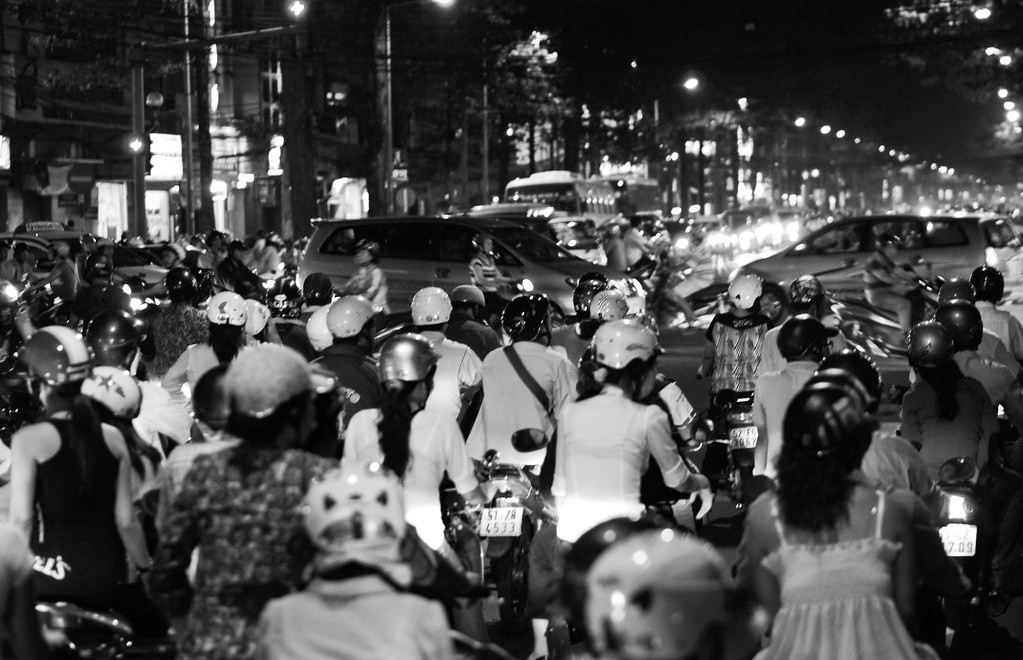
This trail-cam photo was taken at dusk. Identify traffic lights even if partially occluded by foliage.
[286,0,311,56]
[124,135,146,155]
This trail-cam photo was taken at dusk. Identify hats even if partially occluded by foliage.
[819,326,839,339]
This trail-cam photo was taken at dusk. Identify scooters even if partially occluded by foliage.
[443,390,1022,659]
[826,253,945,356]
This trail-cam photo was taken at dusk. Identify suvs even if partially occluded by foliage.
[295,219,649,322]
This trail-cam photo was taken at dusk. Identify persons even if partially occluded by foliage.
[0,216,1023,660]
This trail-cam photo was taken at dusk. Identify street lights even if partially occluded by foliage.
[772,116,957,210]
[654,76,699,166]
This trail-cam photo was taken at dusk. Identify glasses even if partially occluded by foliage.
[816,340,833,349]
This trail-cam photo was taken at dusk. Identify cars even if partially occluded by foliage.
[730,214,1023,314]
[0,230,196,301]
[643,208,837,260]
[463,205,606,266]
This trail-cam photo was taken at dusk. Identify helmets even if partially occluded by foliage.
[783,349,883,460]
[873,233,902,247]
[932,265,1005,350]
[380,286,486,382]
[573,272,629,322]
[80,232,114,283]
[472,232,495,250]
[575,318,660,397]
[189,231,248,256]
[728,273,820,311]
[13,242,29,253]
[906,320,955,368]
[192,343,312,429]
[207,292,271,337]
[563,517,733,660]
[302,273,383,352]
[299,456,407,564]
[502,294,549,341]
[14,311,142,420]
[777,313,825,358]
[48,241,69,260]
[165,266,215,303]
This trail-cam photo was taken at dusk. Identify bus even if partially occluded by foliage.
[504,171,663,220]
